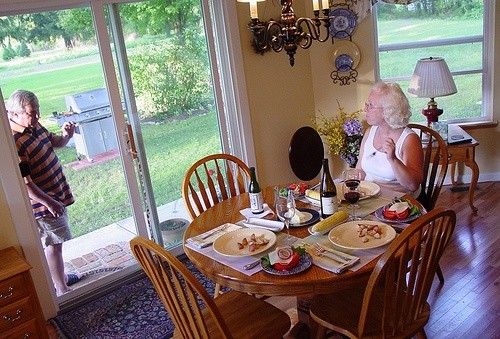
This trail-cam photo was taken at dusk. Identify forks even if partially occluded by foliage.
[202,224,229,240]
[311,242,351,266]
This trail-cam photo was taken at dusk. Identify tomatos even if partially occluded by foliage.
[274,252,299,271]
[382,208,408,219]
[289,182,308,194]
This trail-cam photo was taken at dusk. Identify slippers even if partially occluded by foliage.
[65,273,86,286]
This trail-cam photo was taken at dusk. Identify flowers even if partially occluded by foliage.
[309,99,365,168]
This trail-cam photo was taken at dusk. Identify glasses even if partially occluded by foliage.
[364,101,383,109]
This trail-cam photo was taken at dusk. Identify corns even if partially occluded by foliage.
[312,210,349,233]
[305,189,320,200]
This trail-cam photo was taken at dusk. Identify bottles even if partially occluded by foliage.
[248,167,265,214]
[320,159,338,220]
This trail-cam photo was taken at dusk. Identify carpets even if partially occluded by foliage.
[48,257,230,339]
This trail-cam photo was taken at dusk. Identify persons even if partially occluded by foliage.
[354,80,431,214]
[6,87,85,297]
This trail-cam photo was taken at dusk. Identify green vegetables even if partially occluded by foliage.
[390,196,421,216]
[279,188,289,197]
[261,244,307,268]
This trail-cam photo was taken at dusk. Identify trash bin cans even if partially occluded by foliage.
[65,87,118,157]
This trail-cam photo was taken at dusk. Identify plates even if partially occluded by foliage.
[276,207,320,226]
[337,181,380,200]
[328,220,396,250]
[260,252,313,276]
[374,205,420,223]
[212,228,276,257]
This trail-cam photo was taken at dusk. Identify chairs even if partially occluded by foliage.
[182,154,255,298]
[407,124,444,286]
[130,235,292,339]
[309,207,456,339]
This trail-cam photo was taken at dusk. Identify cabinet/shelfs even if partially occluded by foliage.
[72,117,118,163]
[0,247,48,339]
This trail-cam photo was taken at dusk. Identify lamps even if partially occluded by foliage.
[407,57,457,128]
[237,0,335,67]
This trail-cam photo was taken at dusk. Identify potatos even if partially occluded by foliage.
[237,233,270,253]
[356,223,380,242]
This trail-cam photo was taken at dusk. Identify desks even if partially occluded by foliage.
[183,180,430,338]
[411,125,480,215]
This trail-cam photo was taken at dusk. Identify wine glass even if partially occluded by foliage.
[275,199,298,245]
[342,180,362,220]
[343,169,359,209]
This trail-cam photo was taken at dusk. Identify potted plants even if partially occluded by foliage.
[160,218,189,243]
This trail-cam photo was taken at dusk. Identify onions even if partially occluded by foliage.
[278,248,290,259]
[285,248,294,255]
